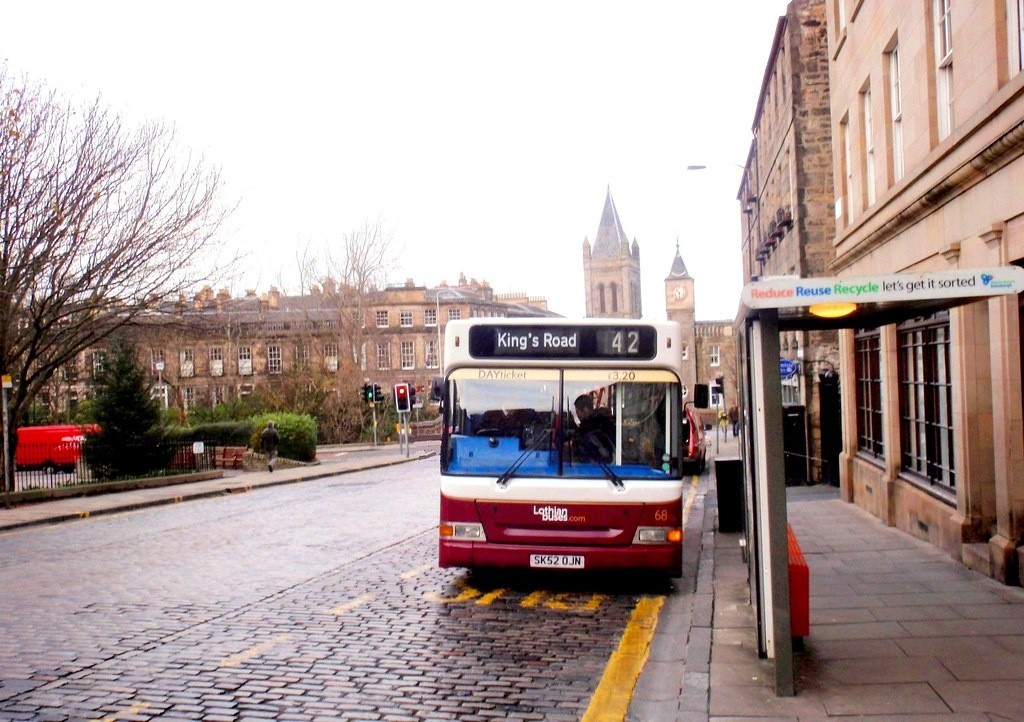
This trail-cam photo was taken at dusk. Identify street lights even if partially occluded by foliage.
[435,288,460,377]
[155,362,166,410]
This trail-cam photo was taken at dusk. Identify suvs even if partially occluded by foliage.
[682,404,713,475]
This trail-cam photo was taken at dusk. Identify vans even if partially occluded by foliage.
[14,424,102,476]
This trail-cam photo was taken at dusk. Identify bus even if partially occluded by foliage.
[426,316,710,579]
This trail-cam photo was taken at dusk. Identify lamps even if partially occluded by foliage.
[687,162,752,179]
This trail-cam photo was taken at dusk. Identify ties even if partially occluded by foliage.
[506,414,511,419]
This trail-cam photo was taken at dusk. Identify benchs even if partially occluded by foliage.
[210,445,249,470]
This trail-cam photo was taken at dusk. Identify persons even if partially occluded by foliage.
[260,421,280,472]
[477,394,530,447]
[565,395,615,463]
[729,401,739,436]
[718,406,729,433]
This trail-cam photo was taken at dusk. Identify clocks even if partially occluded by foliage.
[672,286,686,300]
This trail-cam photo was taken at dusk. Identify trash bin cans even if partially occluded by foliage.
[715,460,744,532]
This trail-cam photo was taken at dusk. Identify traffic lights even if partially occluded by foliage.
[395,383,411,414]
[366,384,374,402]
[715,377,725,394]
[360,382,368,404]
[373,383,385,404]
[408,383,416,412]
